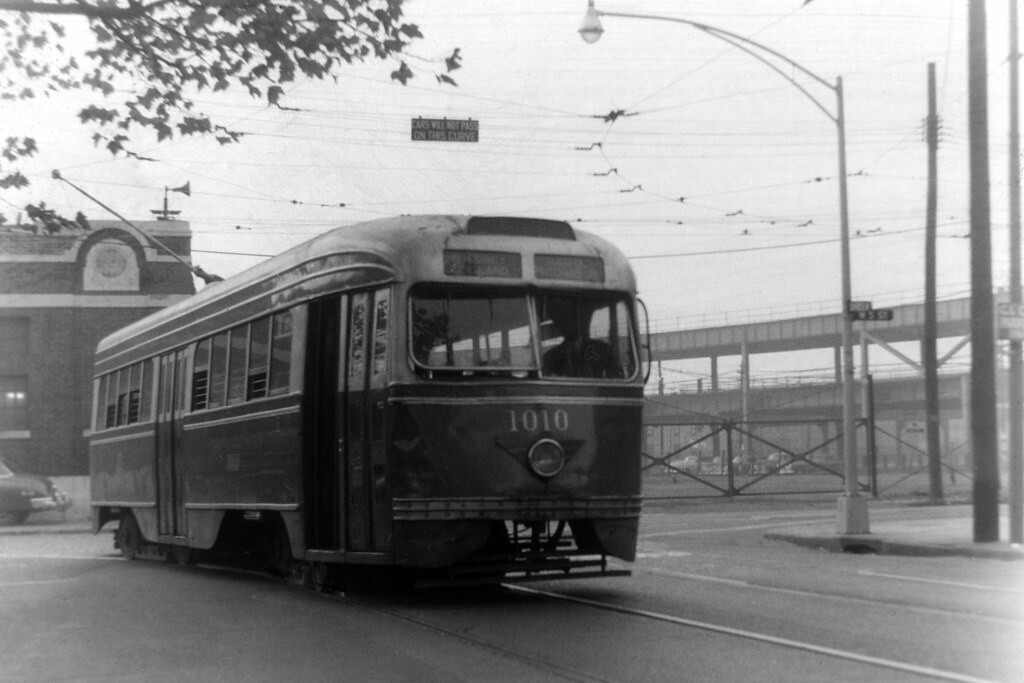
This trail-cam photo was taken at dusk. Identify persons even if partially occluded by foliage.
[541,302,624,378]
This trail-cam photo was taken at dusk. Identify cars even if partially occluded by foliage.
[0,455,70,525]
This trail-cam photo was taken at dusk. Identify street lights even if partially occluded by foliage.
[577,5,873,535]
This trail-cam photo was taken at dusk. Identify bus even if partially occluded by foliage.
[89,211,651,593]
